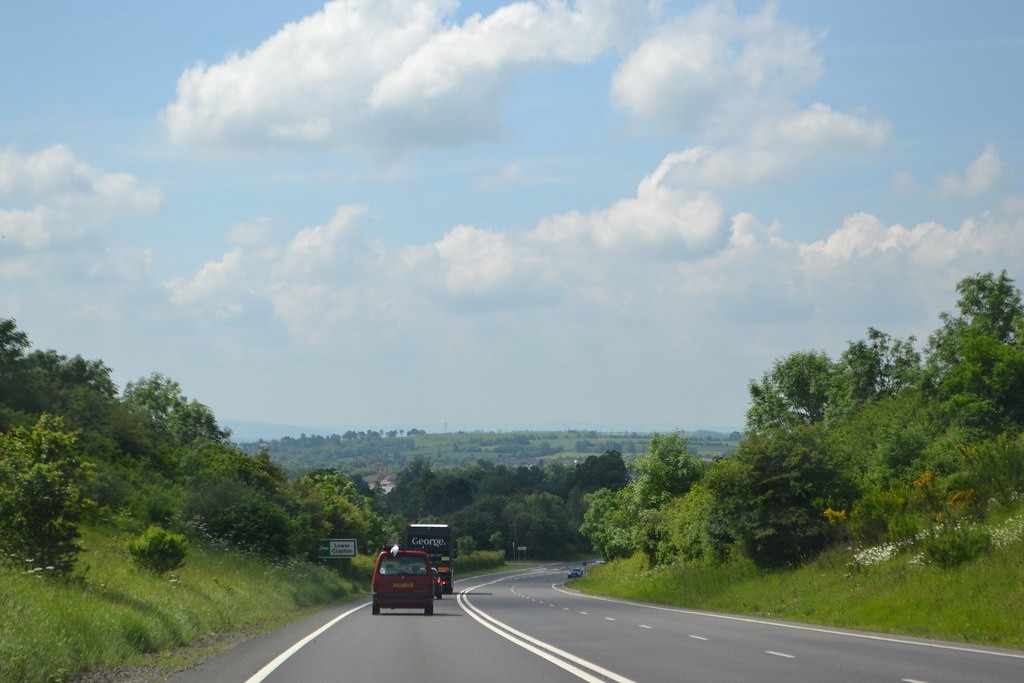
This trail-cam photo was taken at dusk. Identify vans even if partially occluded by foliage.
[367,548,438,616]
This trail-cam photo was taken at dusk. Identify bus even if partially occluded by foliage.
[403,522,458,595]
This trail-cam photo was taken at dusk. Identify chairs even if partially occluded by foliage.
[410,565,423,573]
[384,565,397,574]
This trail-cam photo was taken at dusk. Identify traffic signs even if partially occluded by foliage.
[316,538,357,558]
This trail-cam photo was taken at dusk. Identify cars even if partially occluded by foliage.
[408,567,443,601]
[567,568,583,579]
